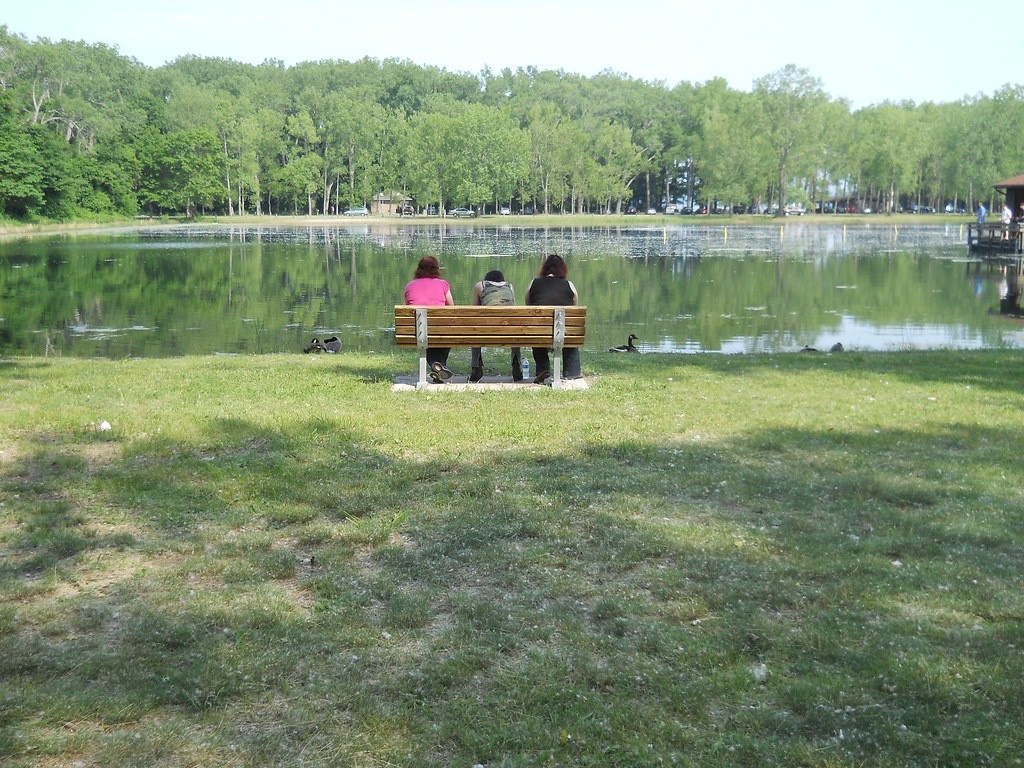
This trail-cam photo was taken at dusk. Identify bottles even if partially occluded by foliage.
[522,358,529,379]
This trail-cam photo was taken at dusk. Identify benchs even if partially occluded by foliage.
[393,303,586,390]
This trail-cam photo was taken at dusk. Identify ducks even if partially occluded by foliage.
[304,336,344,354]
[800,344,816,352]
[609,334,638,352]
[830,343,843,351]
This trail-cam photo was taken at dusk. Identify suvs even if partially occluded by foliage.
[404,206,414,215]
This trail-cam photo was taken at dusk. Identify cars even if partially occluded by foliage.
[500,208,510,215]
[624,204,966,216]
[524,207,533,214]
[448,208,475,217]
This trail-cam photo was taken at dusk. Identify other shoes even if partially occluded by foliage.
[533,370,550,384]
[565,372,583,380]
[468,372,484,383]
[429,361,452,384]
[513,370,523,382]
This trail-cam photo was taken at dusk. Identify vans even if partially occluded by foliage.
[428,207,435,214]
[344,208,369,216]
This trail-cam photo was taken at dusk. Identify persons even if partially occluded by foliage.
[973,201,986,224]
[404,257,454,384]
[524,254,583,383]
[467,270,523,381]
[999,205,1012,240]
[1014,203,1024,237]
